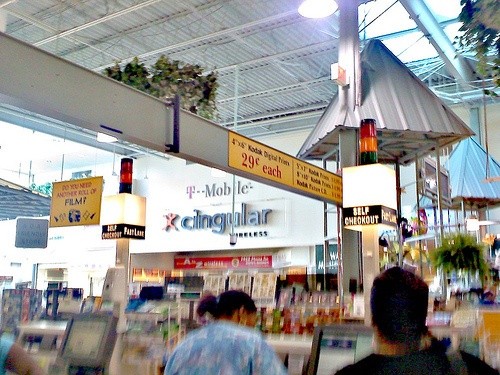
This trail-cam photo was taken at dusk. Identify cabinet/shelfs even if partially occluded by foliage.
[256,291,346,356]
[118,295,180,375]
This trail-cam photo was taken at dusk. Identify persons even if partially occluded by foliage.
[333,266,500,374]
[0,331,47,375]
[162,290,289,374]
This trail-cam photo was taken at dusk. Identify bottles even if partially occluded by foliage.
[272,309,280,332]
[305,314,315,333]
[330,307,340,325]
[261,306,267,331]
[317,305,326,327]
[283,307,291,332]
[292,303,299,332]
